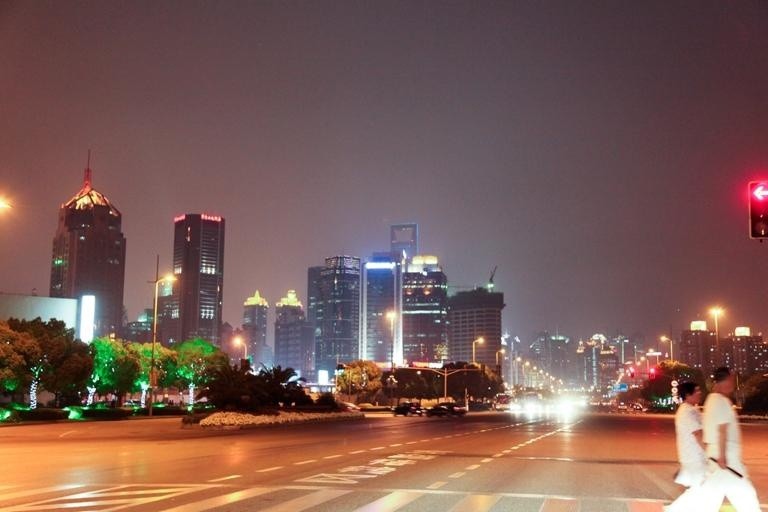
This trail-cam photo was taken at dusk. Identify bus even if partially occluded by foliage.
[496,394,512,410]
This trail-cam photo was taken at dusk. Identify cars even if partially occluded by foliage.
[391,402,422,416]
[426,402,465,417]
[617,403,627,410]
[634,404,642,411]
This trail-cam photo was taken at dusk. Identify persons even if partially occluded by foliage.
[661,385,705,512]
[683,365,762,512]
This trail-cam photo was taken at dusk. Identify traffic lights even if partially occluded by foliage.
[749,181,768,239]
[649,368,656,379]
[631,368,634,377]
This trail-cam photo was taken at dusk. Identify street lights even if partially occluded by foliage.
[496,349,563,392]
[712,307,723,367]
[472,337,484,362]
[384,311,397,405]
[148,274,177,416]
[660,336,673,360]
[234,337,247,359]
